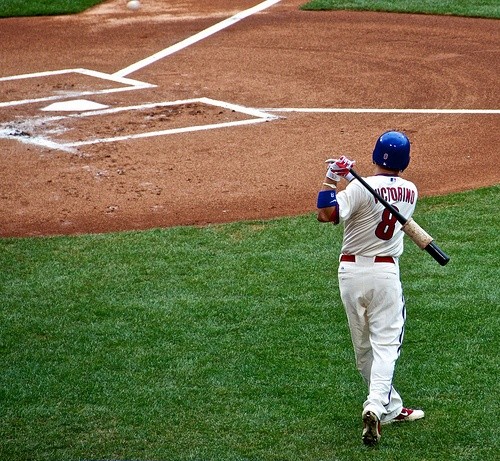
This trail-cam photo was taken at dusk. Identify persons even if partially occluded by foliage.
[317,131,424,445]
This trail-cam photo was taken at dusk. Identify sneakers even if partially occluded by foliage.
[381,407,424,425]
[361,412,380,447]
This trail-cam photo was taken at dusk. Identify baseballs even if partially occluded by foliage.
[126,1,142,11]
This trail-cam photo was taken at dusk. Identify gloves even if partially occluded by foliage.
[326,155,355,182]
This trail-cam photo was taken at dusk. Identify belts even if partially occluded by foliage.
[341,255,394,263]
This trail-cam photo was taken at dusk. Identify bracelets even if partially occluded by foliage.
[322,183,336,189]
[318,191,337,208]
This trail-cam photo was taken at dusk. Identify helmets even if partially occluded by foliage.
[372,131,411,171]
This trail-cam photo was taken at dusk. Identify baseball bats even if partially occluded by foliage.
[351,167,450,266]
